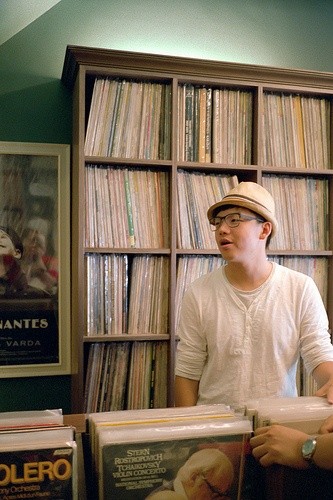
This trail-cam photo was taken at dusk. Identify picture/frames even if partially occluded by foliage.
[0,141,72,378]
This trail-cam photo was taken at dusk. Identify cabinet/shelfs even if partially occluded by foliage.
[60,45,333,414]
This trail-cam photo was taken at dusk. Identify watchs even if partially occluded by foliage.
[302,434,320,468]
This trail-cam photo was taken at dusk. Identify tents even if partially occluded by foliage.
[243,394,333,500]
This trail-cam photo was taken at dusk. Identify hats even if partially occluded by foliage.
[207,181,278,239]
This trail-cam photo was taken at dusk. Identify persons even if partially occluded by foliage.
[251,414,333,469]
[0,225,60,365]
[17,232,60,293]
[143,447,235,500]
[172,181,333,406]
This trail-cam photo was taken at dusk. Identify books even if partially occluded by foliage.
[177,171,239,249]
[85,78,172,160]
[85,341,168,411]
[0,408,78,500]
[87,253,166,332]
[88,403,255,500]
[176,254,227,334]
[177,84,254,165]
[262,172,331,251]
[86,166,167,248]
[263,93,331,169]
[266,255,328,309]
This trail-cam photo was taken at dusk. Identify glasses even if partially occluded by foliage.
[208,211,265,232]
[202,475,229,500]
